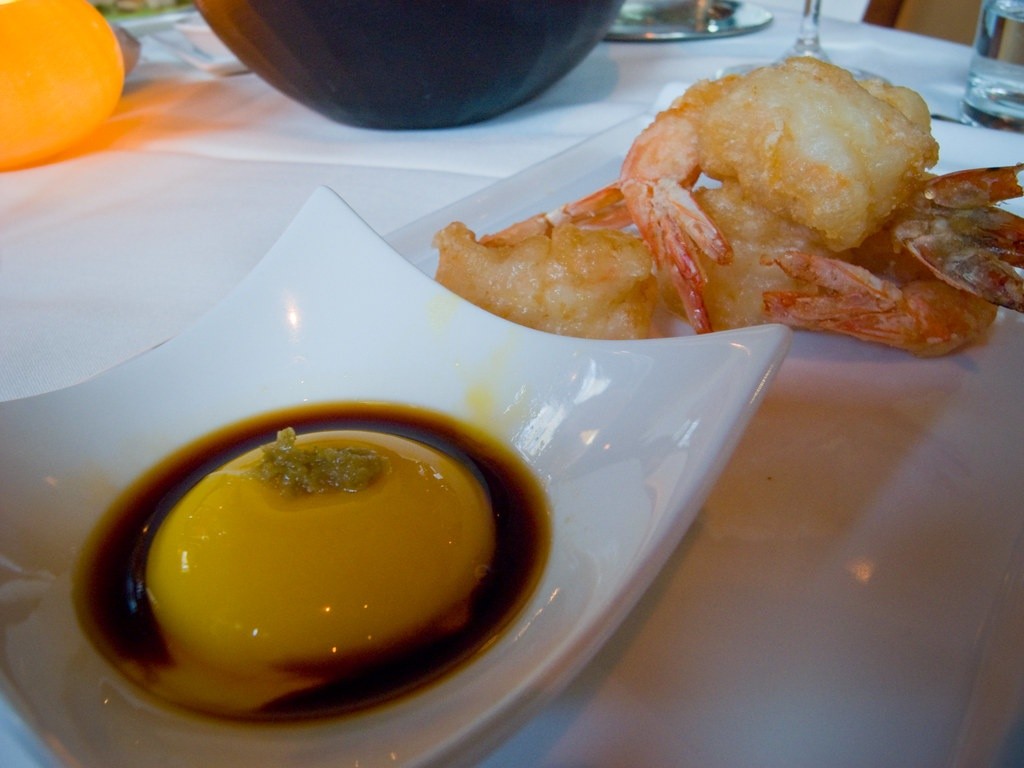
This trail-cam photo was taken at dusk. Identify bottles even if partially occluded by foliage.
[964,0,1024,132]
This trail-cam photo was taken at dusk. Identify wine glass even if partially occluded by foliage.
[716,0,888,81]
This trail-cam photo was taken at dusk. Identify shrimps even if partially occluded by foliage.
[433,51,1024,355]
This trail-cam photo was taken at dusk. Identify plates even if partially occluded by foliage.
[146,35,251,76]
[0,116,1024,768]
[176,15,235,59]
[0,184,794,768]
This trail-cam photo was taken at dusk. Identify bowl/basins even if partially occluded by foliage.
[193,0,627,130]
